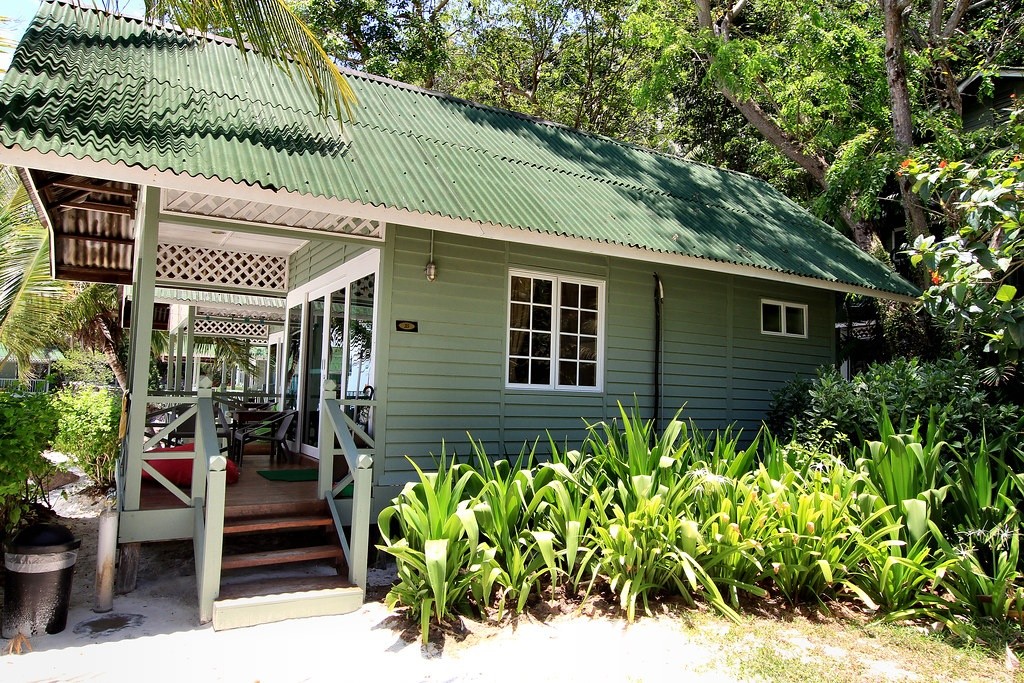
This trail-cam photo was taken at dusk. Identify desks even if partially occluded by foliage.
[232,411,282,464]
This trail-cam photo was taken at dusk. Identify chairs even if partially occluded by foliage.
[234,402,298,467]
[168,404,230,459]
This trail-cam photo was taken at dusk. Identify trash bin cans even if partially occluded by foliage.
[0,524,82,640]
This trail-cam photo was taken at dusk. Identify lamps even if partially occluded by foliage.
[424,262,438,281]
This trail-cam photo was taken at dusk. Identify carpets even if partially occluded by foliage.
[256,468,319,482]
